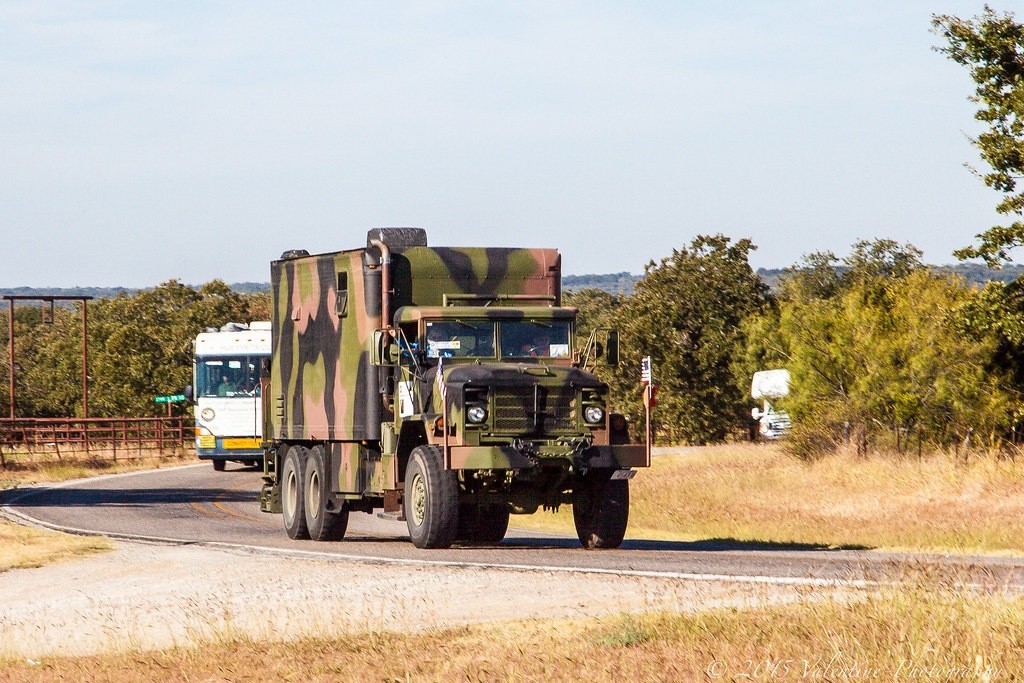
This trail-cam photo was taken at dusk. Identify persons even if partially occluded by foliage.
[217,375,236,397]
[427,325,454,358]
[520,335,551,357]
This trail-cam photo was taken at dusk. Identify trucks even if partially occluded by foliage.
[749,366,795,440]
[263,226,650,548]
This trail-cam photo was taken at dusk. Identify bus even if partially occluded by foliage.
[185,321,274,471]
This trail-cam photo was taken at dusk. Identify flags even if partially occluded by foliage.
[639,354,652,382]
